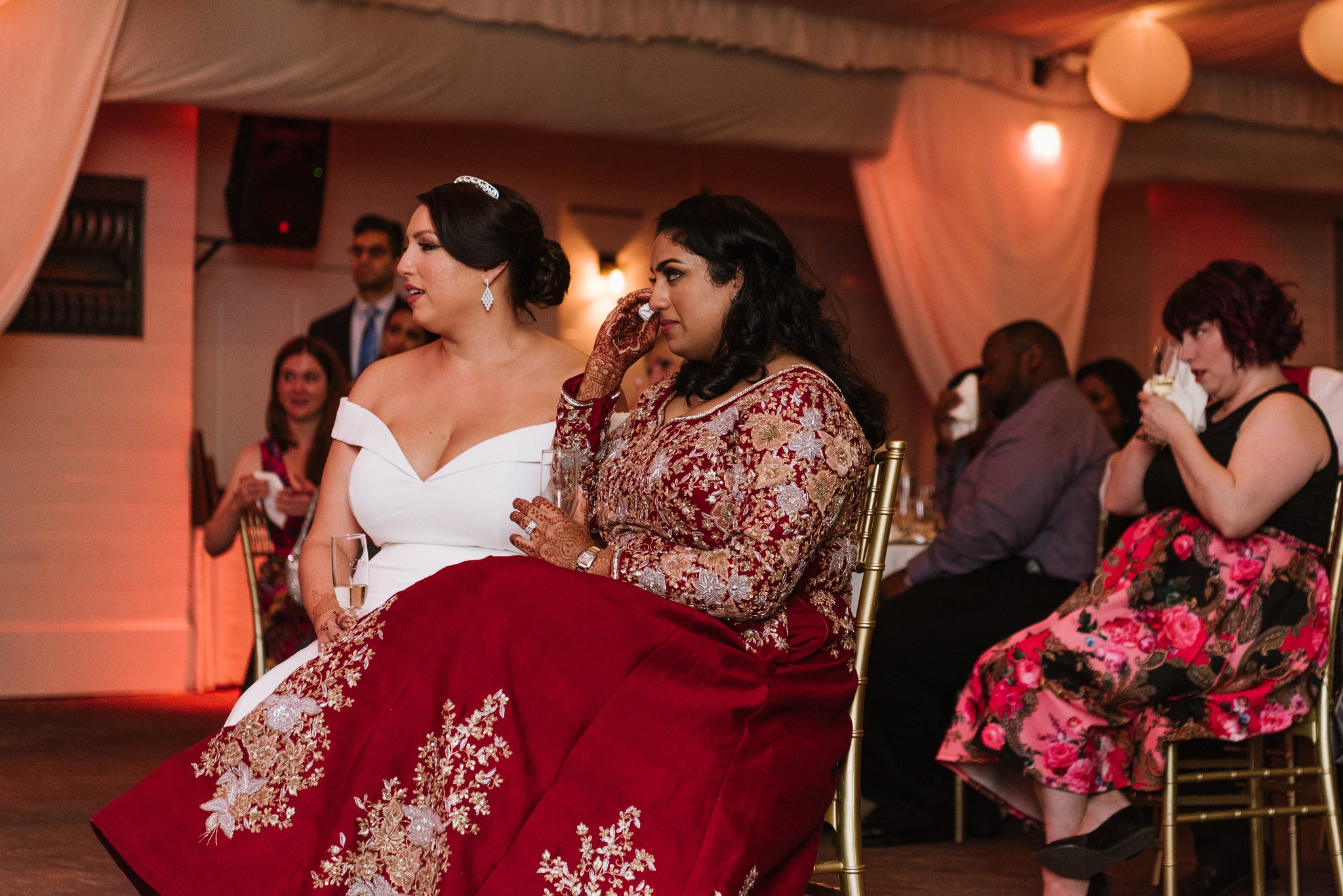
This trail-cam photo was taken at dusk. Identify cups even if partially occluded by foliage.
[541,450,580,517]
[893,473,938,544]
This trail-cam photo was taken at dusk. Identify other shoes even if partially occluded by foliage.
[1085,869,1113,896]
[857,804,954,846]
[1040,804,1155,877]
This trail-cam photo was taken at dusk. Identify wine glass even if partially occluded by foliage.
[331,533,369,622]
[1133,336,1184,446]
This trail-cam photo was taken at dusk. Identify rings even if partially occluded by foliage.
[525,520,537,535]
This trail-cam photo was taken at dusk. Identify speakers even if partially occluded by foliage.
[224,114,332,248]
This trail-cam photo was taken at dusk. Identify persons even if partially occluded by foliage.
[935,258,1339,896]
[306,210,447,381]
[943,362,1012,461]
[221,174,630,729]
[641,338,688,391]
[1075,355,1150,451]
[204,336,356,694]
[373,297,443,369]
[842,316,1115,845]
[86,186,889,896]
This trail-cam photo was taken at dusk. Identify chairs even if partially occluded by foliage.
[956,511,1113,841]
[1153,477,1343,896]
[806,439,907,896]
[241,503,277,681]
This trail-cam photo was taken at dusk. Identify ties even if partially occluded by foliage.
[359,306,381,375]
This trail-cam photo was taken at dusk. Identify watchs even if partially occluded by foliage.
[902,569,913,587]
[575,545,602,573]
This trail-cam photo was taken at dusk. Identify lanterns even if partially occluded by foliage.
[1298,0,1343,87]
[1086,15,1191,122]
